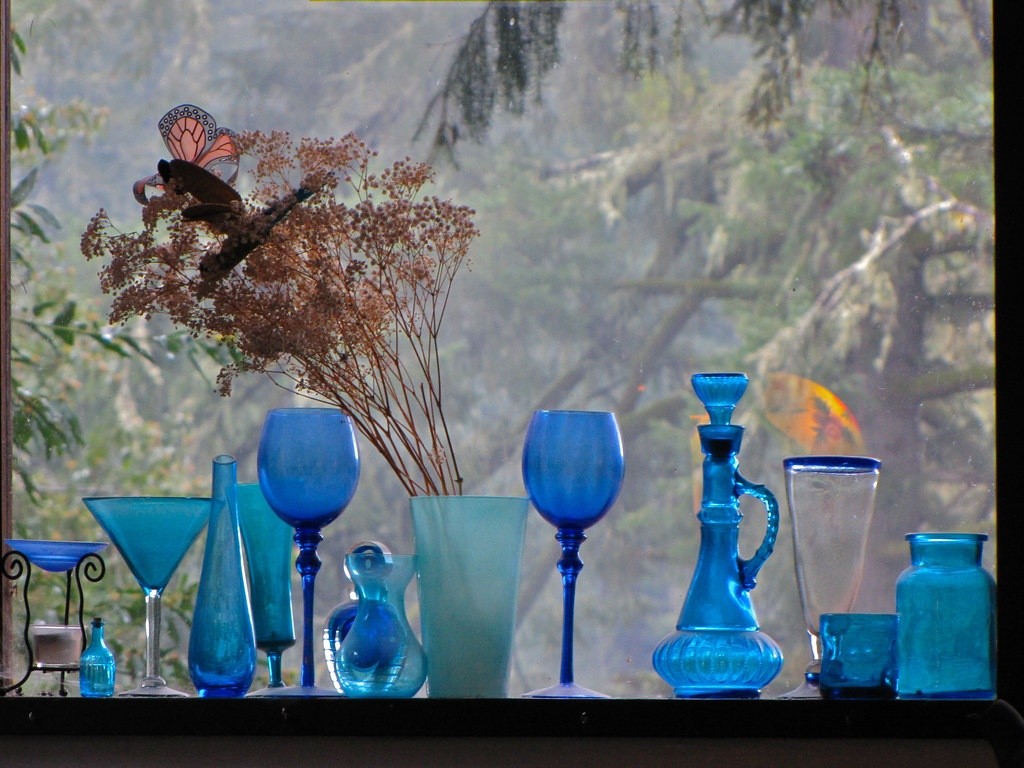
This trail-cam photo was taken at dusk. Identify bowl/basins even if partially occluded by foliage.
[28,626,82,666]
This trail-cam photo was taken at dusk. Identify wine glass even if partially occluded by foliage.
[84,496,209,698]
[223,482,297,688]
[778,457,881,698]
[522,411,627,699]
[246,408,361,698]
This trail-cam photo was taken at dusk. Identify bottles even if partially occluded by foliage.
[186,454,258,701]
[894,533,997,698]
[333,553,427,699]
[79,617,114,697]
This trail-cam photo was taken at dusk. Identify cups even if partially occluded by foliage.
[817,612,896,699]
[409,494,527,699]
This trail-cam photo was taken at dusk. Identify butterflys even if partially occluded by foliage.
[132,103,244,217]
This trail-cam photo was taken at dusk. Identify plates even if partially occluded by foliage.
[4,539,109,572]
